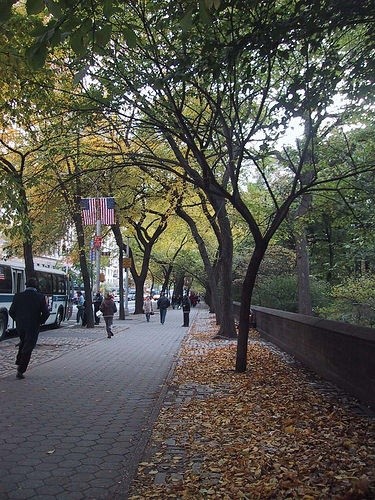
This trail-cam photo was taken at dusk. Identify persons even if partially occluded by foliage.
[157,293,169,324]
[182,295,190,327]
[93,293,103,325]
[75,292,85,325]
[99,293,117,338]
[143,296,154,322]
[189,293,197,307]
[8,277,50,379]
[172,296,183,309]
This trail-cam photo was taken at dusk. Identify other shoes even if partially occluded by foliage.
[109,331,114,336]
[14,360,19,365]
[16,374,25,379]
[108,336,111,338]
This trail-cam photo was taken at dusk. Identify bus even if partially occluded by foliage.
[0,254,74,341]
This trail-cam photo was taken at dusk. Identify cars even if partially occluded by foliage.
[128,290,136,300]
[153,294,160,300]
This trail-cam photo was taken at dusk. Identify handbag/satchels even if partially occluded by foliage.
[96,309,103,318]
[150,311,154,315]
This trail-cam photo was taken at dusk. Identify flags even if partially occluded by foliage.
[80,198,97,226]
[99,198,116,225]
